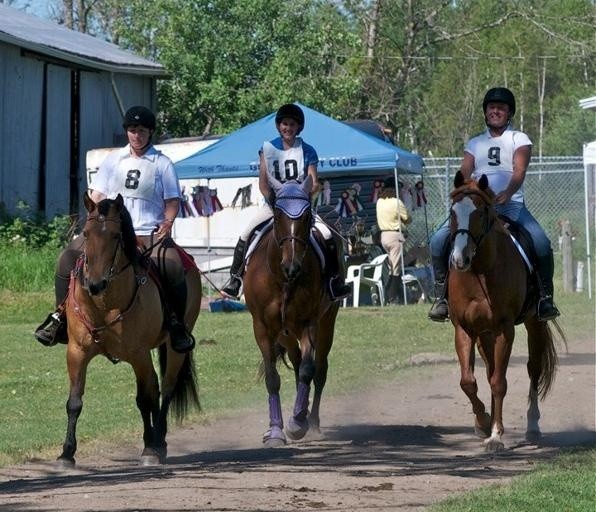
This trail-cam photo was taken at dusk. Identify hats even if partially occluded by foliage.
[383,178,395,188]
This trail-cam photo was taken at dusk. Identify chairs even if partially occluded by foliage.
[343,254,389,309]
[369,246,432,305]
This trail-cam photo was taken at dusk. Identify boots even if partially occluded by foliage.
[223,236,247,301]
[430,256,450,323]
[325,238,351,301]
[37,273,72,343]
[168,280,196,351]
[385,273,403,304]
[534,254,557,319]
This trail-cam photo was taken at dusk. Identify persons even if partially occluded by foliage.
[375,177,411,309]
[219,104,351,308]
[30,106,198,356]
[429,86,562,327]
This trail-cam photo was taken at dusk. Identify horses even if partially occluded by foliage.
[241,170,348,449]
[56,191,204,471]
[445,170,570,456]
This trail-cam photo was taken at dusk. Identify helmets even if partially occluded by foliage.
[123,106,155,132]
[482,88,515,116]
[276,105,305,131]
